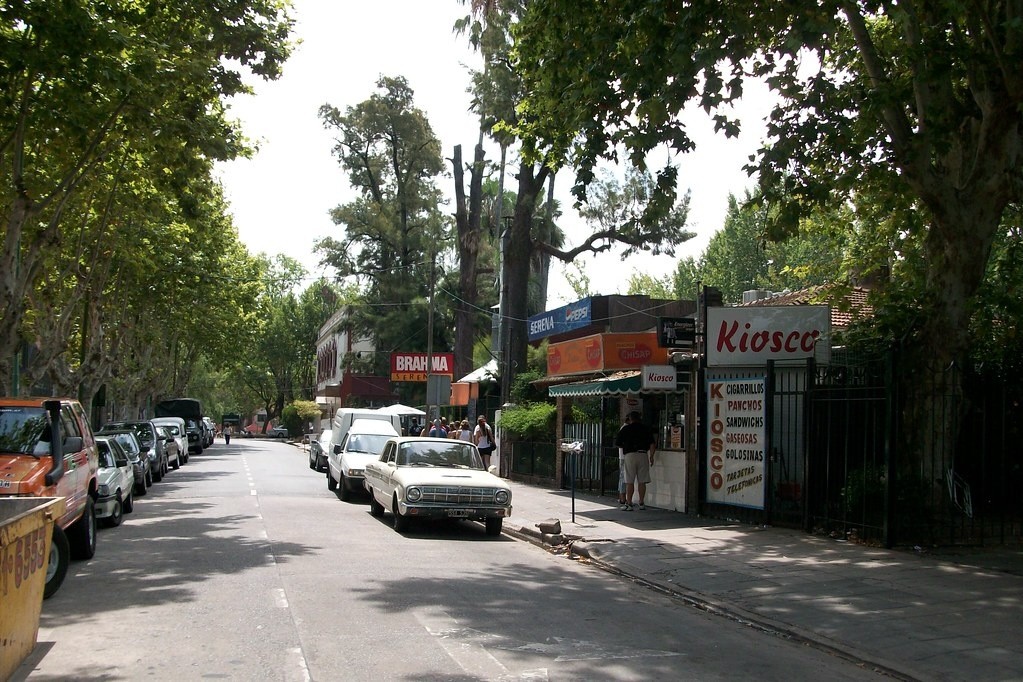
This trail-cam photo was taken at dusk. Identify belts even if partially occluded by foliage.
[638,451,647,454]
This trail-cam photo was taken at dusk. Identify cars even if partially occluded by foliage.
[155,426,181,469]
[202,417,217,448]
[310,430,333,472]
[365,436,514,537]
[92,430,153,495]
[273,425,288,438]
[66,436,135,527]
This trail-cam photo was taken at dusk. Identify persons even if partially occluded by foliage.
[408,415,495,472]
[615,411,656,510]
[223,422,233,445]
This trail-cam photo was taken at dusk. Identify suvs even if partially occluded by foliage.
[101,420,168,482]
[0,398,101,600]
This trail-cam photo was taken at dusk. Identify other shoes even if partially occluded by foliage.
[620,499,627,505]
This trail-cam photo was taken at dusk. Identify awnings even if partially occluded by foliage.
[548,372,691,398]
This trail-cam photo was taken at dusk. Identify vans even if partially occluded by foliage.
[149,417,190,465]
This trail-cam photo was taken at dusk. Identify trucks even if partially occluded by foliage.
[327,405,427,503]
[154,398,205,454]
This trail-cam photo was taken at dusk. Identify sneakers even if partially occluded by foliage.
[622,504,633,511]
[638,503,645,510]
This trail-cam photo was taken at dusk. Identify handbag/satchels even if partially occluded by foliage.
[490,442,496,451]
